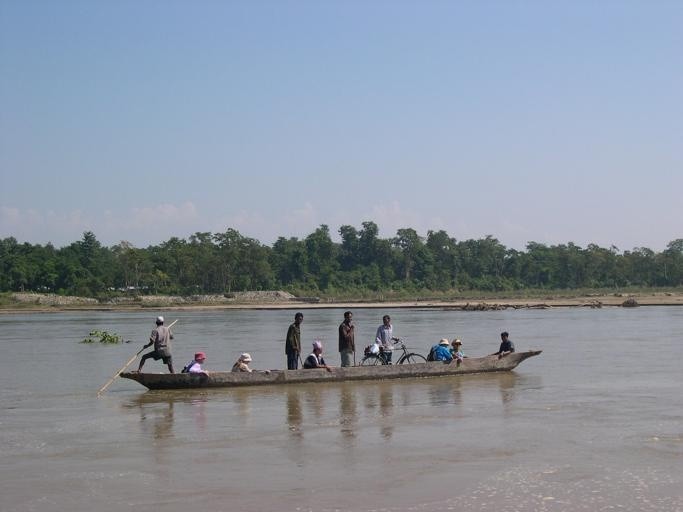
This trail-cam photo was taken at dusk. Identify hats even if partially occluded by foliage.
[157,316,164,323]
[438,339,449,345]
[313,340,322,349]
[195,352,206,360]
[451,338,462,345]
[239,353,252,361]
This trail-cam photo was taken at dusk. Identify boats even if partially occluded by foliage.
[117,349,547,393]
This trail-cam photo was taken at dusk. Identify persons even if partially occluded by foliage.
[449,339,464,362]
[488,332,515,359]
[339,311,355,367]
[285,312,303,371]
[181,352,209,377]
[430,339,453,361]
[131,317,175,374]
[231,353,253,372]
[304,340,332,372]
[375,315,398,365]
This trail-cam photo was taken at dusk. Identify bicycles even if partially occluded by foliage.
[358,335,429,366]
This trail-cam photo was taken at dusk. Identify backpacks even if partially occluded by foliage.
[427,346,439,361]
